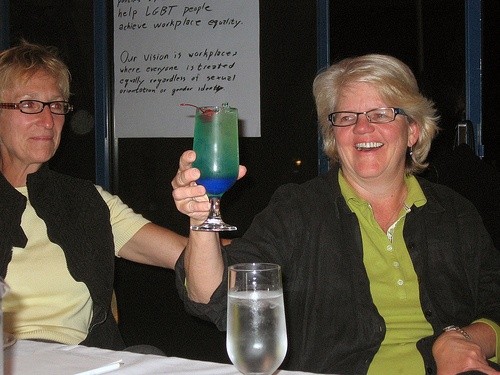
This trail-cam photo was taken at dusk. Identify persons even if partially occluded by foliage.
[171,55,500,375]
[0,44,233,356]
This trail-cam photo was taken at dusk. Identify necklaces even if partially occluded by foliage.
[382,195,404,232]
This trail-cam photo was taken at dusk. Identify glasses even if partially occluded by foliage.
[328,107,408,127]
[1,99,73,115]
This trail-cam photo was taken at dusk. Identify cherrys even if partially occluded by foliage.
[179,103,214,123]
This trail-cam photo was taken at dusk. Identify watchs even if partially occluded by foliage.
[442,325,473,342]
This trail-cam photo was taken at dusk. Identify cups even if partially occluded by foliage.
[226,263,288,375]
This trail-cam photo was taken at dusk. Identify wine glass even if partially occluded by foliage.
[189,104,240,232]
